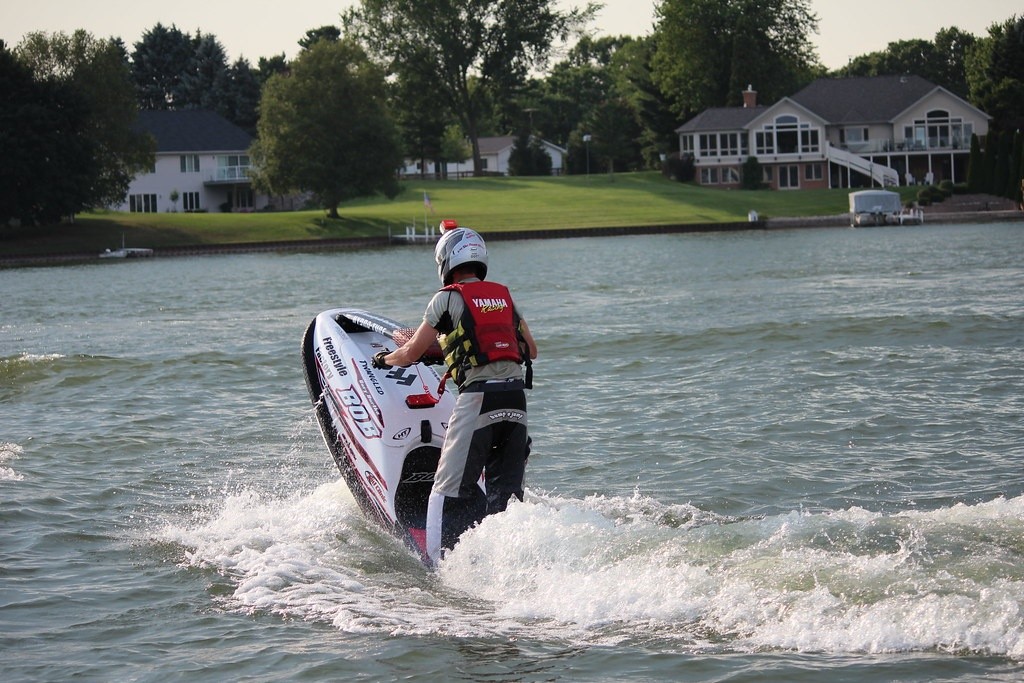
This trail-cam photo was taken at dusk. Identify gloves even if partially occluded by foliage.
[373,350,393,370]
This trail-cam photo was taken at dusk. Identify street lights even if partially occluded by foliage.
[583,135,592,178]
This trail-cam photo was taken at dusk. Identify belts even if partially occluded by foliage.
[461,380,523,393]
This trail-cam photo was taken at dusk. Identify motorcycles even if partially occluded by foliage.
[300,307,525,573]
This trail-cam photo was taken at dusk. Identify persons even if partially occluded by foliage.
[370,218,538,567]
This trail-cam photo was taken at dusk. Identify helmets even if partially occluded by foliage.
[435,220,488,287]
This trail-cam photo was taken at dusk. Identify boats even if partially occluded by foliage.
[98,248,154,258]
